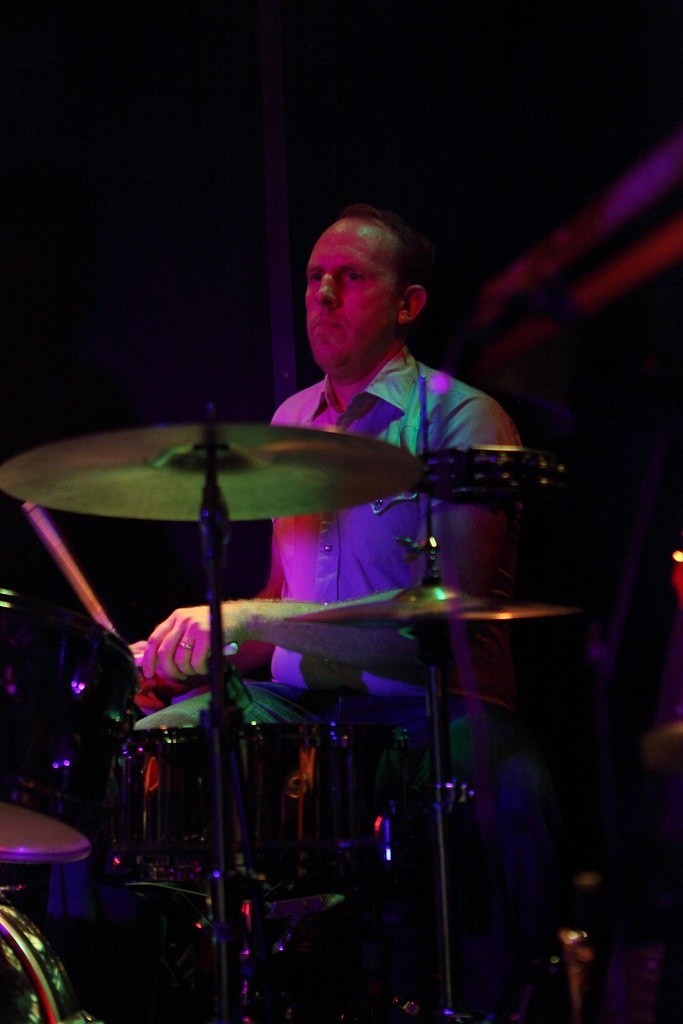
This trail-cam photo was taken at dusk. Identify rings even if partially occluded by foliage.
[178,642,194,650]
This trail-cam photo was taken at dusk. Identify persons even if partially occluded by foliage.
[128,203,556,1024]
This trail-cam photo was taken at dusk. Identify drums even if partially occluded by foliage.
[0,585,137,866]
[116,721,414,893]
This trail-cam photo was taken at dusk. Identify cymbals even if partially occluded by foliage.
[0,416,424,522]
[281,580,590,626]
